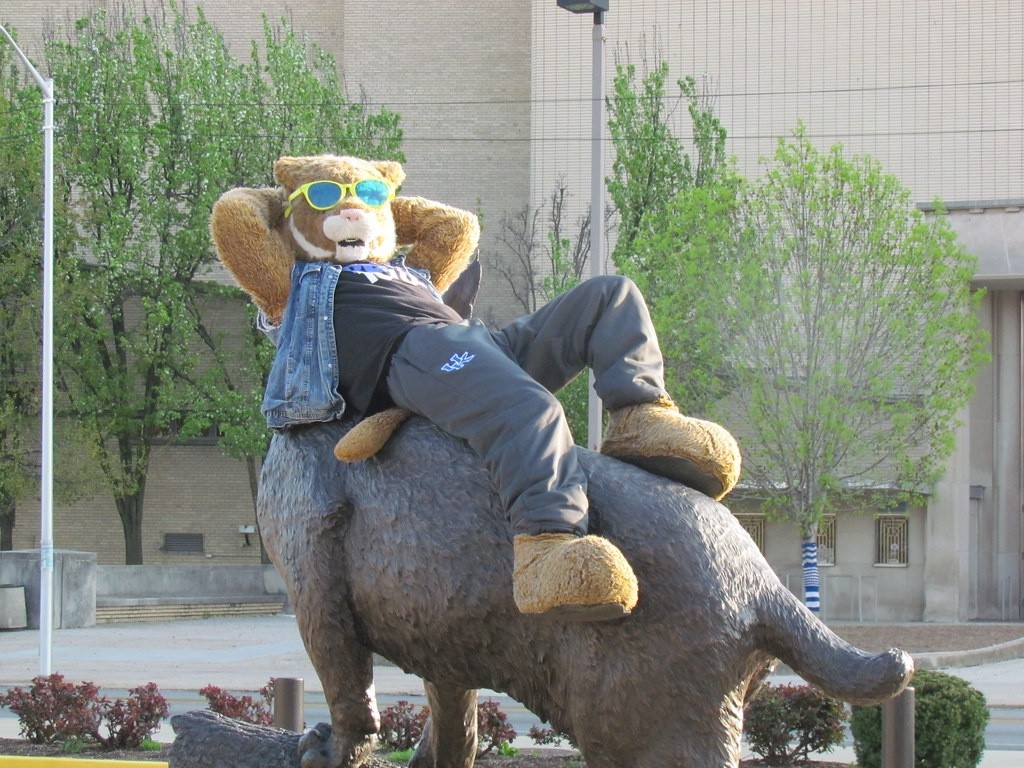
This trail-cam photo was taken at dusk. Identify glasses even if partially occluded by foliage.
[286,179,393,211]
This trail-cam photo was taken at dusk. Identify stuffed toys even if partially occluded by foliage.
[208,152,741,615]
